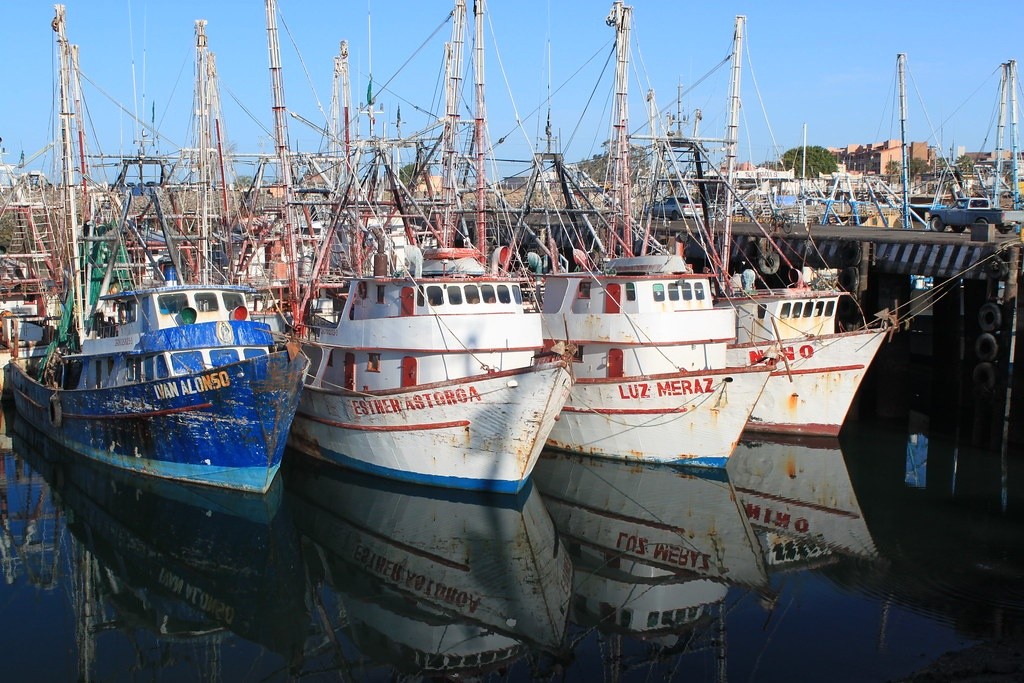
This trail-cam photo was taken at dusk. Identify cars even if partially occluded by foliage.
[109,179,155,187]
[645,196,705,220]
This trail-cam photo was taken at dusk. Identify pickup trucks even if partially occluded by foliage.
[928,197,1024,236]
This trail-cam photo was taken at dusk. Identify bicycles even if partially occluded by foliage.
[770,211,792,235]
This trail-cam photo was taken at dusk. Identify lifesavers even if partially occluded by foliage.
[976,334,997,361]
[759,252,780,274]
[985,254,1008,279]
[979,303,1002,331]
[973,362,996,391]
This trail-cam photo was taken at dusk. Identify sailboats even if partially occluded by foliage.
[0,1,1023,495]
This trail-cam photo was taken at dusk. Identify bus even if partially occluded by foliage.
[701,168,796,203]
